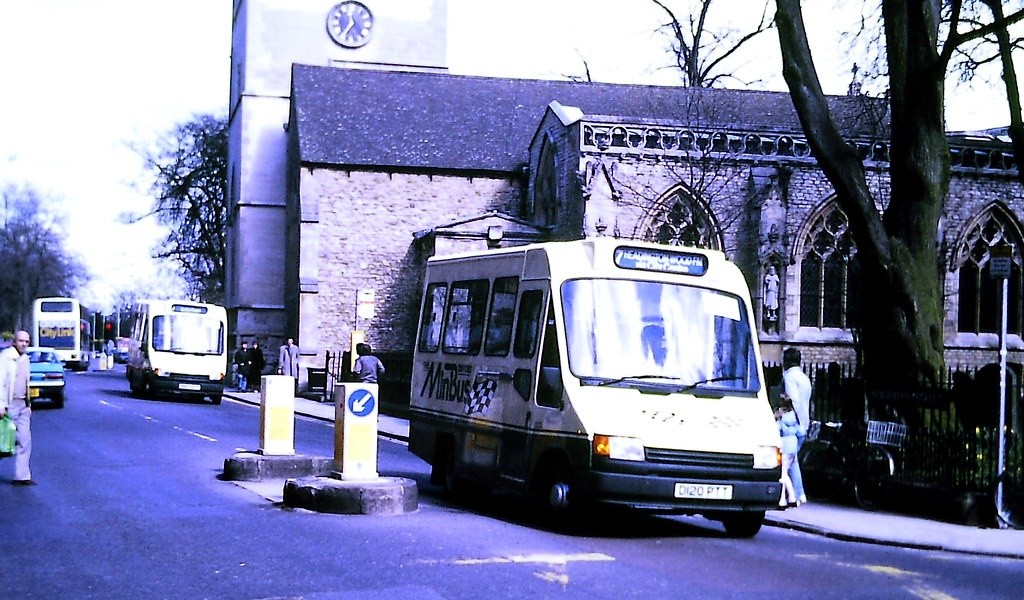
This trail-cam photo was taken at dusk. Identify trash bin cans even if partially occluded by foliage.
[306,366,328,393]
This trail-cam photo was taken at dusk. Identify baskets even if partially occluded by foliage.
[865,420,909,447]
[805,419,831,445]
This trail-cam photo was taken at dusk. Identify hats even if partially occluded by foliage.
[781,347,802,363]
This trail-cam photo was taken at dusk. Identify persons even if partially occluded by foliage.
[247,341,266,393]
[235,342,254,393]
[775,393,805,511]
[0,331,37,486]
[278,337,300,398]
[782,348,812,504]
[354,343,385,422]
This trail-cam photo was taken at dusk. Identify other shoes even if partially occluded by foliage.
[12,479,37,485]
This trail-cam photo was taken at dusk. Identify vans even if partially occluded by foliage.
[406,234,785,540]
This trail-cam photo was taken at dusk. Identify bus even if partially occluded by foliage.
[30,296,95,372]
[125,299,229,407]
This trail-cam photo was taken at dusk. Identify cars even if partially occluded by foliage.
[25,345,66,409]
[95,336,129,364]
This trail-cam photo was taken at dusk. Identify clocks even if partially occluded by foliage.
[325,0,374,48]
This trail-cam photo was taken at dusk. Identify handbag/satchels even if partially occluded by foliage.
[0,412,17,453]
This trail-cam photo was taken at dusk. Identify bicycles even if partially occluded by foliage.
[990,458,1024,532]
[797,417,908,512]
